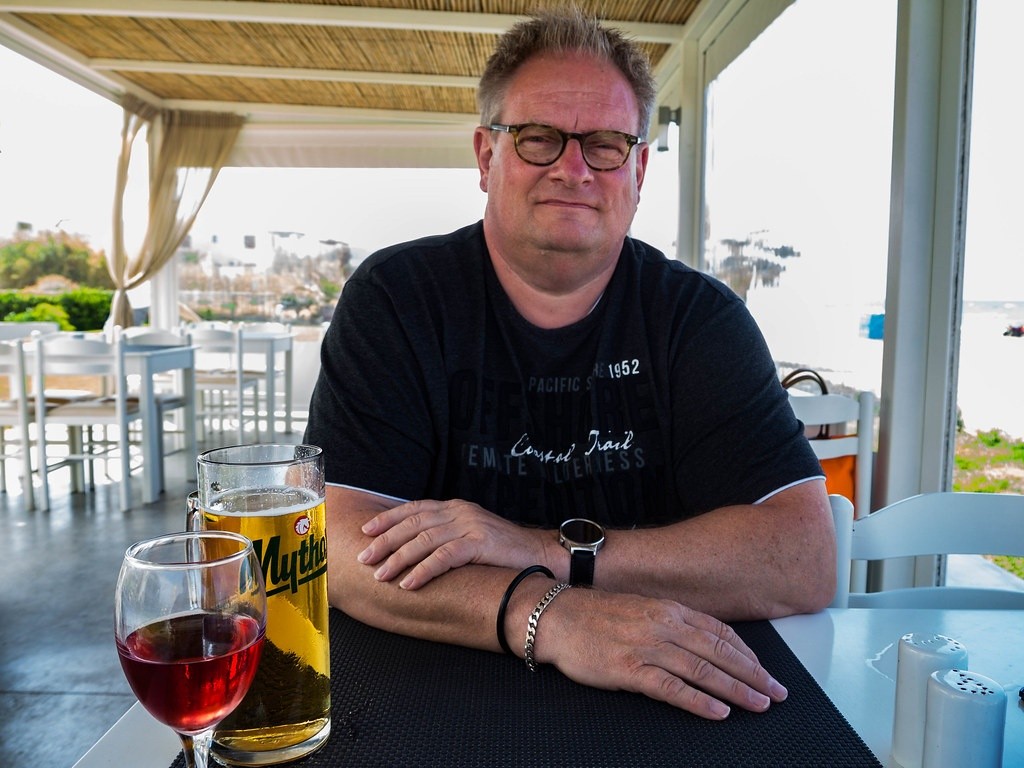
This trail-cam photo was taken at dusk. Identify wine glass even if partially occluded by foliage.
[111,530,270,768]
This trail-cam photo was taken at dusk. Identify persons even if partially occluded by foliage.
[284,6,836,720]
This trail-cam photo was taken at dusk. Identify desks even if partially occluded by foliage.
[65,607,1024,768]
[192,334,297,446]
[7,342,245,512]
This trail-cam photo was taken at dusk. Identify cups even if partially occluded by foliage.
[186,443,333,768]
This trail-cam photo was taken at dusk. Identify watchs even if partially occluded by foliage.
[558,518,608,589]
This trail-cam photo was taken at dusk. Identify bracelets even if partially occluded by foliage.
[497,565,556,660]
[524,583,571,672]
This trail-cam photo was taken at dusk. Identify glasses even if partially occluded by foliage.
[490,123,641,171]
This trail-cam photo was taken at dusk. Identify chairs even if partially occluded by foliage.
[828,493,1024,608]
[0,320,298,513]
[789,390,876,594]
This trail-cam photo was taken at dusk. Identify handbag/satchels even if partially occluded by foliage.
[781,369,855,521]
